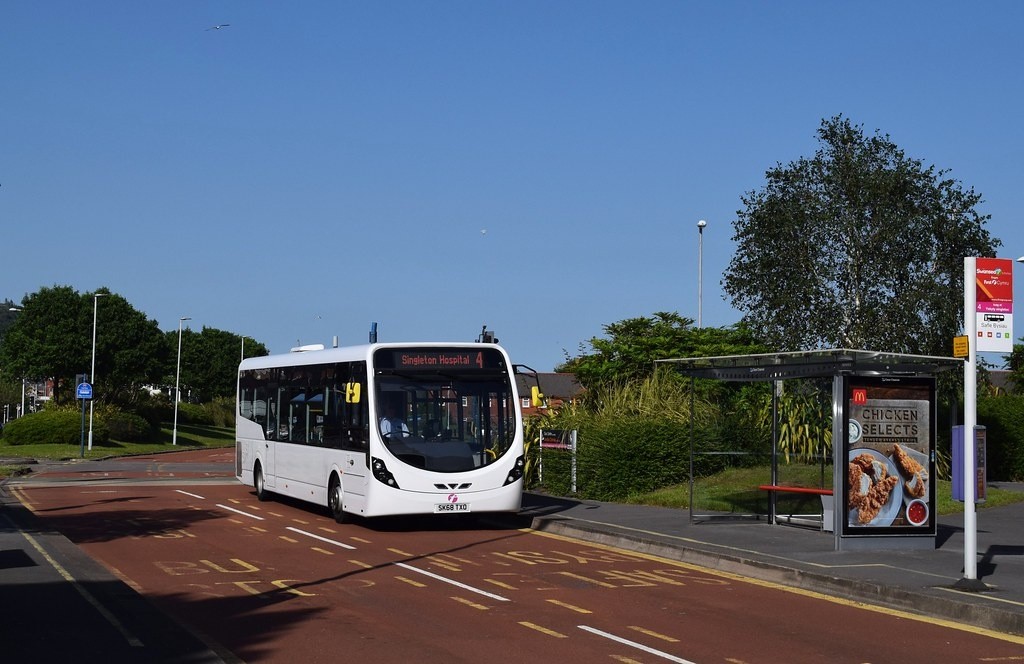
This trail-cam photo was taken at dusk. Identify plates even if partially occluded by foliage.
[848,447,903,527]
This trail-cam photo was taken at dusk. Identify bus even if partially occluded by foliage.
[236,340,547,523]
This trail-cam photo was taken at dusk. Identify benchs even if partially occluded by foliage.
[758,483,833,533]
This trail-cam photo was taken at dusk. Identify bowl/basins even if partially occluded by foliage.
[906,499,929,526]
[849,419,862,445]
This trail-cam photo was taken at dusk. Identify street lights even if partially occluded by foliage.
[86,293,110,451]
[169,316,193,446]
[697,219,708,327]
[9,307,25,419]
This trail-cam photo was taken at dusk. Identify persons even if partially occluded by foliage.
[310,423,321,444]
[279,421,289,441]
[379,400,409,439]
